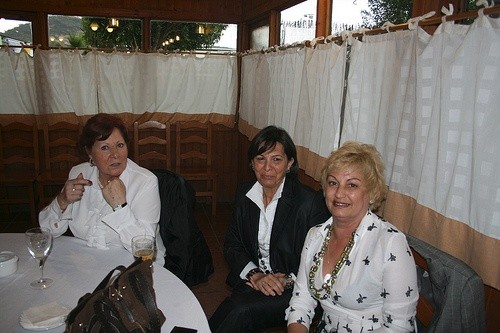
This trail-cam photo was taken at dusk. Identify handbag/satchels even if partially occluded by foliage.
[63,255,167,333]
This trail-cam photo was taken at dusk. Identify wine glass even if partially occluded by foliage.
[25,227,55,289]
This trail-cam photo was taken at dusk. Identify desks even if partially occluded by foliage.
[0,233,212,333]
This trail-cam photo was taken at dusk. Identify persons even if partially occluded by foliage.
[39,112,166,268]
[208,126,332,333]
[284,142,420,333]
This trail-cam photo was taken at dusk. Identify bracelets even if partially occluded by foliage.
[285,274,294,288]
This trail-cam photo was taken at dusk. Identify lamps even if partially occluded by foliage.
[195,24,204,34]
[89,20,100,31]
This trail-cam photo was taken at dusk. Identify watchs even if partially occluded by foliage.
[113,202,127,211]
[246,268,264,280]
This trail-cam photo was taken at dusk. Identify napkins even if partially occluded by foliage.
[18,302,69,329]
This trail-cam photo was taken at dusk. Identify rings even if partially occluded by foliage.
[72,188,76,192]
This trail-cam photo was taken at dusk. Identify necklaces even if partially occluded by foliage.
[309,225,357,301]
[98,180,104,189]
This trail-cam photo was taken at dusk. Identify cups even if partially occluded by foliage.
[131,235,155,263]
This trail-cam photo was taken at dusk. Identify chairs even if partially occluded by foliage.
[36,117,83,210]
[406,236,464,333]
[134,120,172,171]
[176,120,217,216]
[0,117,38,229]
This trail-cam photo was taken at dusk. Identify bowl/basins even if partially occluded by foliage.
[0,250,18,278]
